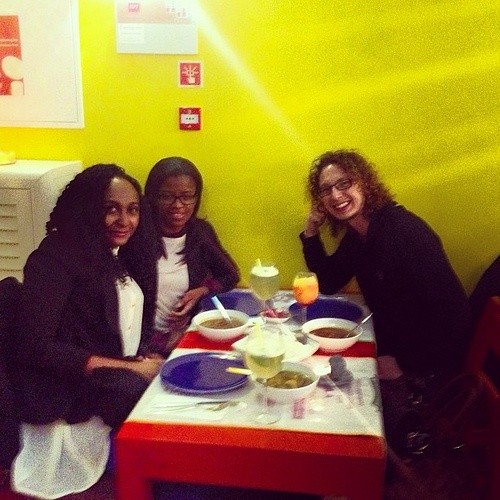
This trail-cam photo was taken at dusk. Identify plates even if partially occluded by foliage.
[231,321,321,363]
[199,292,274,316]
[161,351,248,396]
[288,298,364,324]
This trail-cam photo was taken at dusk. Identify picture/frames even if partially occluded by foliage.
[0,0,84,130]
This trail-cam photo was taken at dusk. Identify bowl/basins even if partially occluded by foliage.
[301,317,363,353]
[250,361,320,405]
[192,309,250,342]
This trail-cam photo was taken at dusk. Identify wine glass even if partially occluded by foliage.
[293,271,319,324]
[245,333,285,426]
[250,267,279,318]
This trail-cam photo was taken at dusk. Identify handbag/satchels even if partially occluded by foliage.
[91,356,148,430]
[403,371,482,474]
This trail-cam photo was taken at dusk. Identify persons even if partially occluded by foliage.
[4,158,169,500]
[297,147,471,411]
[117,156,242,366]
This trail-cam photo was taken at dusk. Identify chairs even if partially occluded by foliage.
[458,295,500,500]
[469,255,500,331]
[0,277,25,469]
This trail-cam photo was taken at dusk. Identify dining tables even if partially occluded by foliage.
[112,285,388,500]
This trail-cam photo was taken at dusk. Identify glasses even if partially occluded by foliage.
[153,187,198,205]
[317,174,357,199]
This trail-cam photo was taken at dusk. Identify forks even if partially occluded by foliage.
[207,400,234,411]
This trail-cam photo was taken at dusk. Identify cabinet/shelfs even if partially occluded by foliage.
[0,160,82,284]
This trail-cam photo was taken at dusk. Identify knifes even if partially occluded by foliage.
[196,399,240,407]
